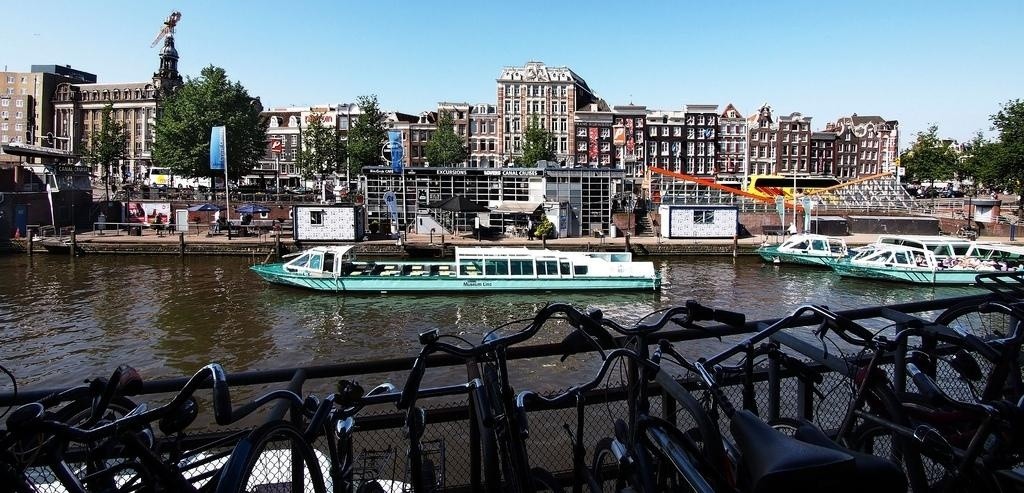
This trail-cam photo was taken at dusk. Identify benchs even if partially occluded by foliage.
[203,219,292,237]
[761,225,790,235]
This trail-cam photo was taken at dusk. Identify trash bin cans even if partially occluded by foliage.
[98,214,107,230]
[611,223,617,238]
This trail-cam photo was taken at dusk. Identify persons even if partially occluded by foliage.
[154,212,161,234]
[166,211,175,236]
[133,203,143,216]
[212,208,222,233]
[786,221,798,236]
[526,216,534,240]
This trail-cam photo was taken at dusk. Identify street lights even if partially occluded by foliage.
[122,184,135,235]
[966,184,973,226]
[384,189,390,233]
[626,188,632,231]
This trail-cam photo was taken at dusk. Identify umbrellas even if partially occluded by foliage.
[186,202,226,223]
[233,203,271,225]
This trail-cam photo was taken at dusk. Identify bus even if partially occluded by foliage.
[740,173,843,207]
[141,165,224,188]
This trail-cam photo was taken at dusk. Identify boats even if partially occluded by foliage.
[248,244,666,294]
[758,233,1023,284]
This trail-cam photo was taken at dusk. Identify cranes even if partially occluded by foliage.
[149,8,182,49]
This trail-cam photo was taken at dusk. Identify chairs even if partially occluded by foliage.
[350,265,481,278]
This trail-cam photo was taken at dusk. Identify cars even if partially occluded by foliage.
[233,184,315,195]
[907,186,992,198]
